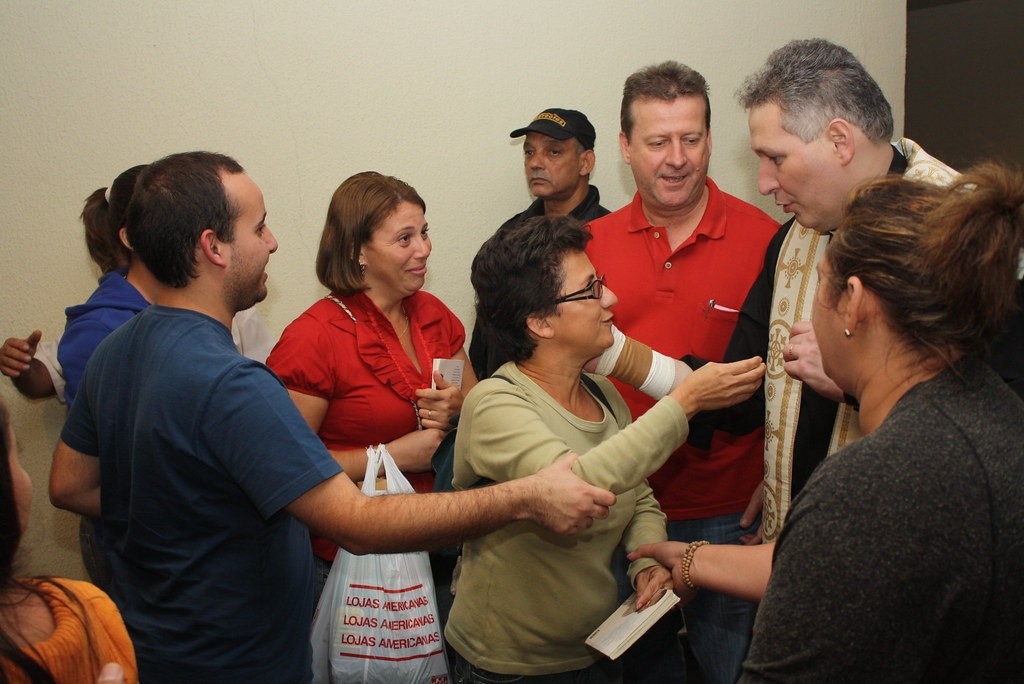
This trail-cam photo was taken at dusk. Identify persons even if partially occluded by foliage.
[444,213,767,684]
[48,150,615,684]
[469,108,612,381]
[0,399,138,684]
[57,163,162,588]
[627,161,1024,684]
[583,39,971,546]
[579,62,785,684]
[265,172,475,592]
[1,306,276,409]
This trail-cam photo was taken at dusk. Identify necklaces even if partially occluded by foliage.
[398,317,410,338]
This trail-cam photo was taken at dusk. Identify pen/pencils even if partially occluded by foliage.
[709,299,715,308]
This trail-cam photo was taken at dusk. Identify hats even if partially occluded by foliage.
[510,108,596,152]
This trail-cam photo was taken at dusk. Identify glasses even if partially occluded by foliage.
[544,275,606,307]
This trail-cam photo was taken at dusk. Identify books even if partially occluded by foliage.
[431,358,465,393]
[584,588,680,661]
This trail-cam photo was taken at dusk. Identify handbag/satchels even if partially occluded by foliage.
[309,443,452,684]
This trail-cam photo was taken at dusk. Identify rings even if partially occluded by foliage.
[428,410,432,419]
[788,344,795,359]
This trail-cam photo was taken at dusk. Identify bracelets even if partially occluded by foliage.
[682,540,710,588]
